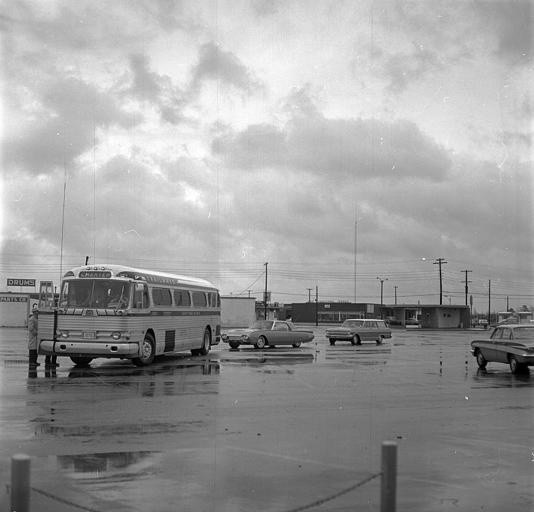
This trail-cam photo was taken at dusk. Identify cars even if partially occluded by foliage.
[386,316,397,325]
[324,319,391,346]
[470,324,534,373]
[405,318,420,326]
[222,320,314,350]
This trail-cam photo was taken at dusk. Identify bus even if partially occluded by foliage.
[37,265,222,365]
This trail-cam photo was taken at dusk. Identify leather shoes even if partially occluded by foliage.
[29,362,40,365]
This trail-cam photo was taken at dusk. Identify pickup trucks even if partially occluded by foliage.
[472,319,488,329]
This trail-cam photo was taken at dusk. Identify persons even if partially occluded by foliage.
[27,302,41,368]
[44,353,59,366]
